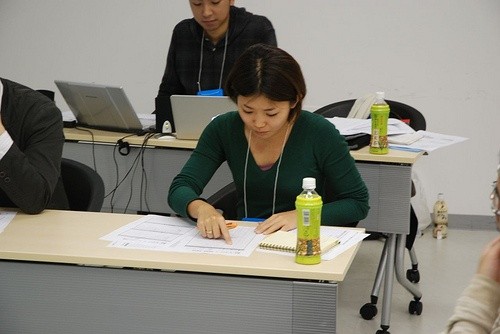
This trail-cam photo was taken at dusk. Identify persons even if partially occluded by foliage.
[0,77,70,215]
[444,165,500,334]
[152,0,278,134]
[167,44,371,245]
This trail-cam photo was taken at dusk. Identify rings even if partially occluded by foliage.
[205,230,213,233]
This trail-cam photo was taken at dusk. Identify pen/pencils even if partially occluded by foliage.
[220,222,238,234]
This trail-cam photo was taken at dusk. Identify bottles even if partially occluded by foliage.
[432,193,448,239]
[295,177,323,265]
[369,92,390,154]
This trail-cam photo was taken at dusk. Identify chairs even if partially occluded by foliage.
[61,158,106,212]
[312,97,426,323]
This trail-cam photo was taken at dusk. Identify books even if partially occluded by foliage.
[330,116,470,152]
[257,229,340,256]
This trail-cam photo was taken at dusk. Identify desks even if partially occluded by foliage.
[0,208,365,334]
[62,127,425,334]
